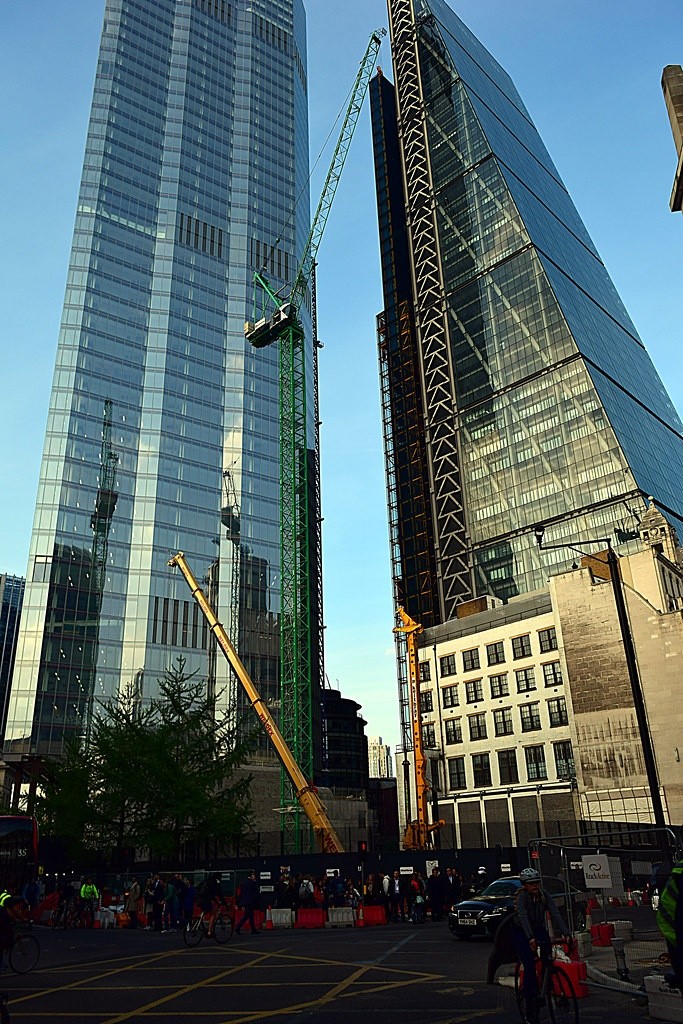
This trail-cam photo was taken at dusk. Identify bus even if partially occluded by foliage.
[0,815,40,895]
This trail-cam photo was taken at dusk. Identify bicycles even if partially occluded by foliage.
[183,901,234,948]
[51,900,99,930]
[0,919,40,974]
[515,940,579,1024]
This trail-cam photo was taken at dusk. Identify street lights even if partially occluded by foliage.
[534,526,669,847]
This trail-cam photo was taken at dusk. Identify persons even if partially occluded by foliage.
[201,872,231,937]
[515,868,572,1024]
[0,882,28,971]
[657,857,683,993]
[278,866,488,924]
[235,870,262,934]
[124,871,197,933]
[22,877,121,927]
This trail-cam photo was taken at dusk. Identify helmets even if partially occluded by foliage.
[520,868,540,882]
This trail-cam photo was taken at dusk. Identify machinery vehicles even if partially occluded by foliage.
[394,605,446,849]
[170,552,346,852]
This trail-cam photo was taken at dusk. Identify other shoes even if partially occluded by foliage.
[141,926,150,930]
[234,928,242,935]
[251,931,261,935]
[208,932,216,938]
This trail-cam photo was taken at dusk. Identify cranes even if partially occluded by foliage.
[241,26,388,852]
[72,397,120,755]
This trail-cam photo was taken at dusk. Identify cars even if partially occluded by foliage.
[448,876,586,940]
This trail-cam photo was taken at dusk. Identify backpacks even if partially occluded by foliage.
[513,886,546,911]
[299,881,311,898]
[194,880,208,896]
